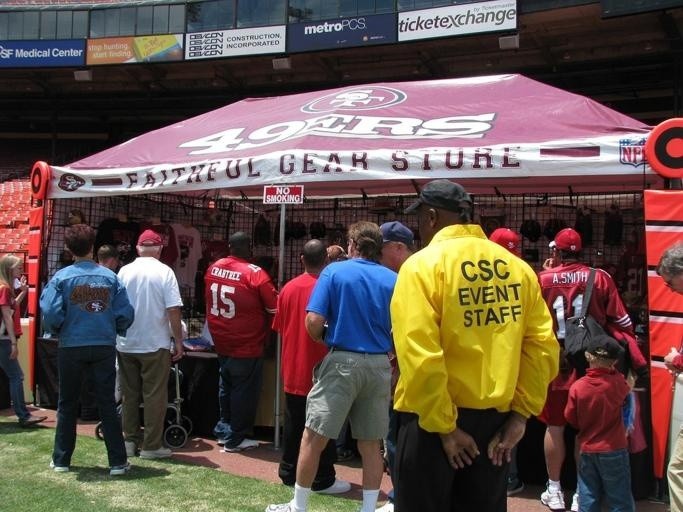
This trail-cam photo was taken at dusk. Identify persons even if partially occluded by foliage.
[271,179,646,512]
[656,245,682,512]
[1,220,182,477]
[203,231,277,452]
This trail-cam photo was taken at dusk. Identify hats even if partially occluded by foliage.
[137,229,164,247]
[377,221,414,246]
[399,179,477,216]
[546,227,583,255]
[584,334,628,359]
[488,228,523,256]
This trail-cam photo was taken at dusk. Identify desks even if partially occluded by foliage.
[35,334,284,438]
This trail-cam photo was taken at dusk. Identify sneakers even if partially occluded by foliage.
[314,478,352,495]
[334,445,356,461]
[123,441,137,457]
[540,486,579,512]
[18,412,49,427]
[136,445,173,459]
[222,438,260,453]
[264,501,306,512]
[108,459,132,475]
[49,459,70,472]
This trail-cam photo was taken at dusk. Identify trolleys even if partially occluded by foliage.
[92,342,201,447]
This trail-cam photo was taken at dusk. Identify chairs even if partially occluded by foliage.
[0,177,35,259]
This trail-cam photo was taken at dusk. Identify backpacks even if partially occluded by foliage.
[563,312,625,368]
